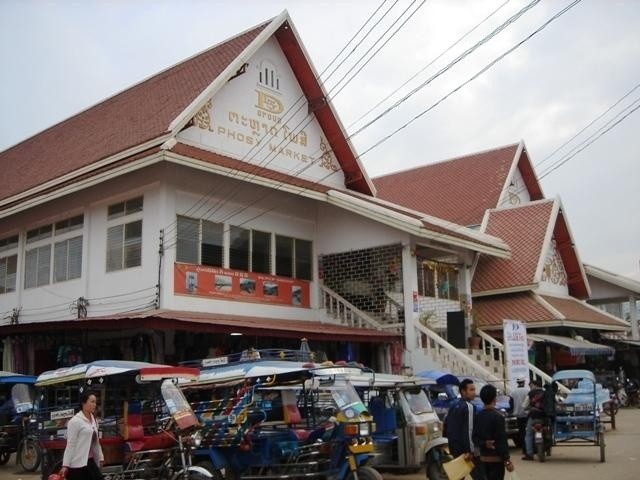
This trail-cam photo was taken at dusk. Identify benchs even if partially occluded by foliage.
[555,415,595,425]
[43,436,123,466]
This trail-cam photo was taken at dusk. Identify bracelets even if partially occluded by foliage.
[505,461,511,465]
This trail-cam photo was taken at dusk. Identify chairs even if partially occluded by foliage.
[368,396,399,443]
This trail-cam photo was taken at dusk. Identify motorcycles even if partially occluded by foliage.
[0,346,611,479]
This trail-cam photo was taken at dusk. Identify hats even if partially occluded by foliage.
[516,375,526,382]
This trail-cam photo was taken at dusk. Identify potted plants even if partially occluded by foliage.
[419,307,435,347]
[468,309,481,349]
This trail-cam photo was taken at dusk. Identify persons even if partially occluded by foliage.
[442,378,478,480]
[470,384,515,480]
[520,379,554,460]
[510,376,538,455]
[56,390,106,480]
